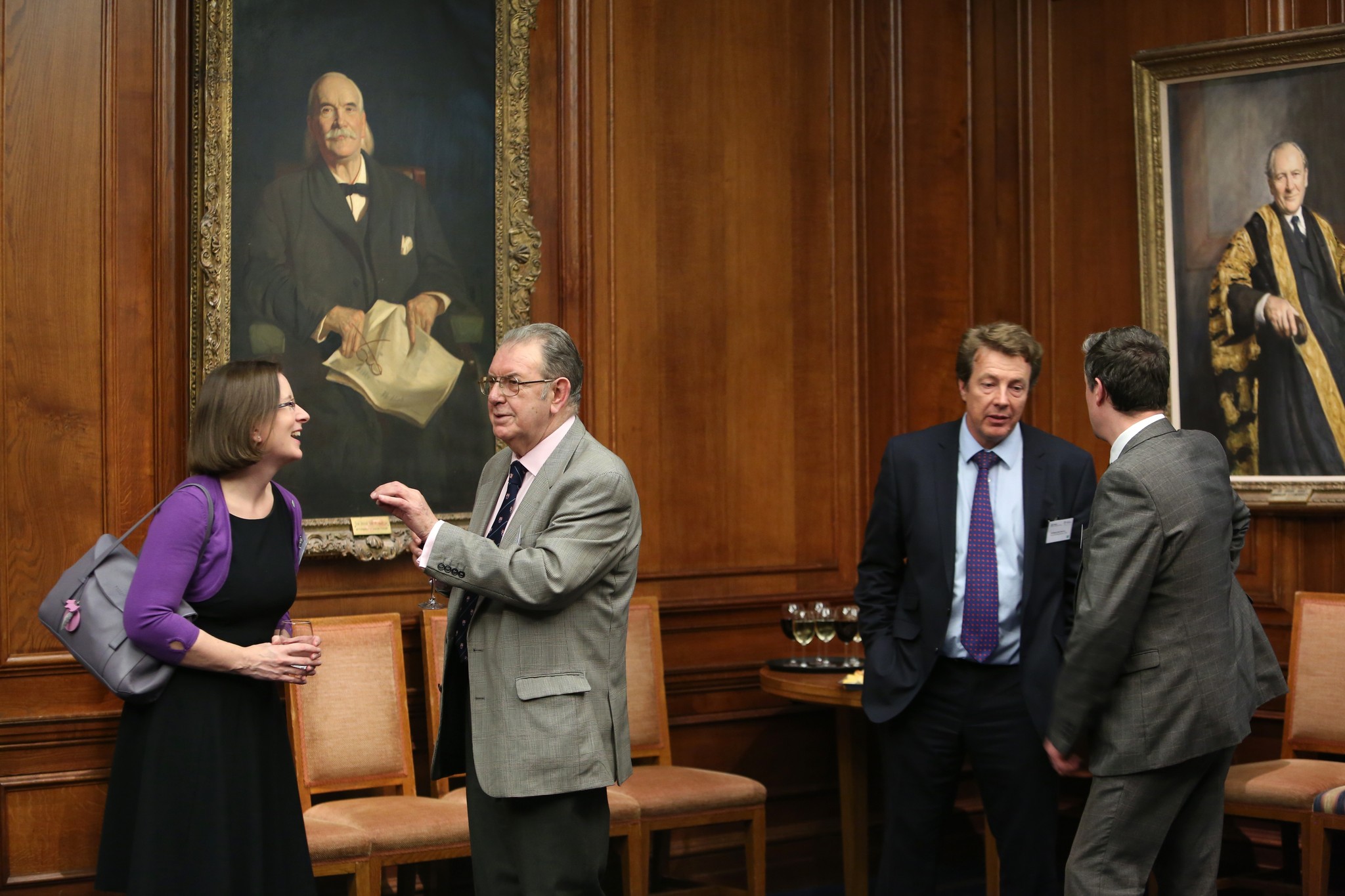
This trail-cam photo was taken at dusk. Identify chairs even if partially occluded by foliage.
[280,597,764,896]
[1221,598,1345,896]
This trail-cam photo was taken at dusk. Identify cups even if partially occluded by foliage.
[280,620,313,677]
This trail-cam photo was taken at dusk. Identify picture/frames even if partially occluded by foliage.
[184,0,542,575]
[1130,24,1345,511]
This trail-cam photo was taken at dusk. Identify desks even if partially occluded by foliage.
[767,664,870,896]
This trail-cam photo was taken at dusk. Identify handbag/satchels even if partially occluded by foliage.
[36,483,216,706]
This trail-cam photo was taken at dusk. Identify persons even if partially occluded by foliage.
[95,364,324,896]
[854,320,1097,896]
[1042,324,1289,896]
[370,322,643,896]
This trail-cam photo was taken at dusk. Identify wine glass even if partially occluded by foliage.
[812,607,837,668]
[808,600,833,666]
[833,604,861,669]
[780,603,804,667]
[791,609,816,668]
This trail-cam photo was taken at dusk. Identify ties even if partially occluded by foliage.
[452,460,529,664]
[959,450,1001,662]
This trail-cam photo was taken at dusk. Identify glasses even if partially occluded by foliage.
[272,398,296,411]
[477,375,555,398]
